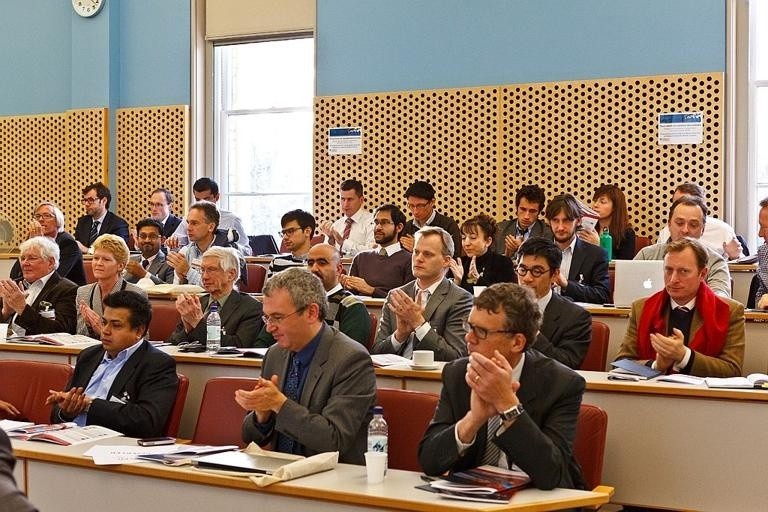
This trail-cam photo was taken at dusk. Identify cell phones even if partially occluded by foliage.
[137,437,176,446]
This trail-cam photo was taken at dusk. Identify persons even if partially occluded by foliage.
[252,243,371,348]
[369,226,475,362]
[416,281,588,491]
[262,179,636,304]
[0,400,21,417]
[169,246,264,348]
[629,182,768,311]
[44,290,179,439]
[125,178,251,293]
[75,233,151,342]
[610,236,745,376]
[516,236,593,370]
[9,201,86,291]
[72,182,129,256]
[1,427,38,511]
[2,235,78,339]
[233,267,377,466]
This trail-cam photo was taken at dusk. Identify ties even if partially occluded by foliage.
[340,218,353,257]
[89,222,100,248]
[277,355,301,454]
[142,260,149,269]
[480,413,501,467]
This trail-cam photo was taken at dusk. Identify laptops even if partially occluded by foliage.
[615,260,666,309]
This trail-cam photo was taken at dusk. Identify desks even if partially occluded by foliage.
[131,277,768,370]
[0,423,621,512]
[10,328,768,506]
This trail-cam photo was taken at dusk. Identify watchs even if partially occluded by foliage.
[500,404,524,420]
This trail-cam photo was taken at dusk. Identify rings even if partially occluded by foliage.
[473,375,480,383]
[399,305,403,311]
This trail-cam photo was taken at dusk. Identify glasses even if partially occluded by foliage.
[33,214,55,220]
[262,305,307,326]
[515,266,550,278]
[81,198,100,205]
[278,228,301,237]
[462,321,517,340]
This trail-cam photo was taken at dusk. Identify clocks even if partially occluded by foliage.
[72,1,104,17]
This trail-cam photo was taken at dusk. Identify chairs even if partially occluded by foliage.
[585,320,618,372]
[357,382,436,472]
[1,357,76,422]
[362,311,390,348]
[241,262,269,293]
[189,371,280,452]
[558,404,617,485]
[157,370,191,431]
[141,300,189,336]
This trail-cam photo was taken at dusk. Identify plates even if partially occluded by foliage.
[409,364,442,369]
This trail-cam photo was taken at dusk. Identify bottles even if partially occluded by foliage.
[205,305,221,354]
[599,226,612,262]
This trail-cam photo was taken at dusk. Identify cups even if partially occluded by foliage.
[367,406,389,477]
[413,350,433,365]
[472,285,487,297]
[364,451,387,485]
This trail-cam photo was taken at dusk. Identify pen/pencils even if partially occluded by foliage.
[607,375,640,382]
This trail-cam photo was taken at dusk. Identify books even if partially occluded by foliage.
[704,372,768,390]
[575,194,599,230]
[6,421,125,446]
[137,277,206,299]
[419,463,533,504]
[6,332,103,349]
[609,359,661,380]
[137,436,176,447]
[369,354,411,367]
[191,450,298,475]
